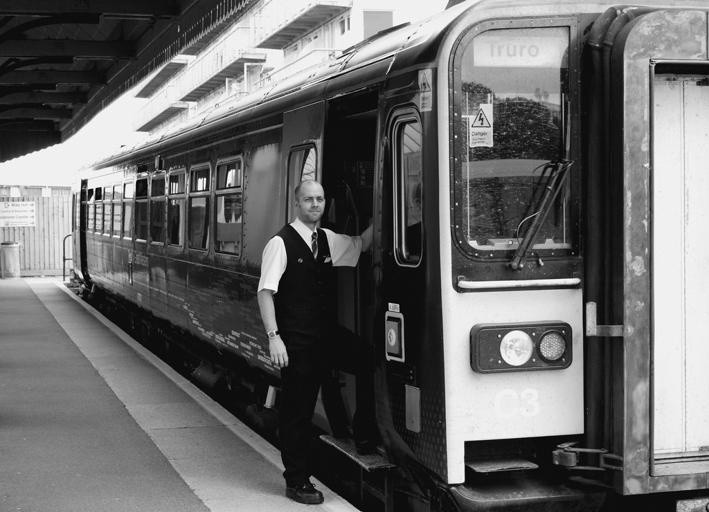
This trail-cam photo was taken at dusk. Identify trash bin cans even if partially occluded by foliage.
[0,242,20,278]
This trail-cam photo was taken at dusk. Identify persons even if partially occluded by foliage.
[251,176,387,506]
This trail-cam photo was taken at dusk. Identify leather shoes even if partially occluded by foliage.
[286,479,324,504]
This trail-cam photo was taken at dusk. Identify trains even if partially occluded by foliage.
[62,0,709,512]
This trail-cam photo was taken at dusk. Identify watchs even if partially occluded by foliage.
[265,330,280,339]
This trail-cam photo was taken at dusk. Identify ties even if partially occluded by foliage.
[311,232,318,259]
